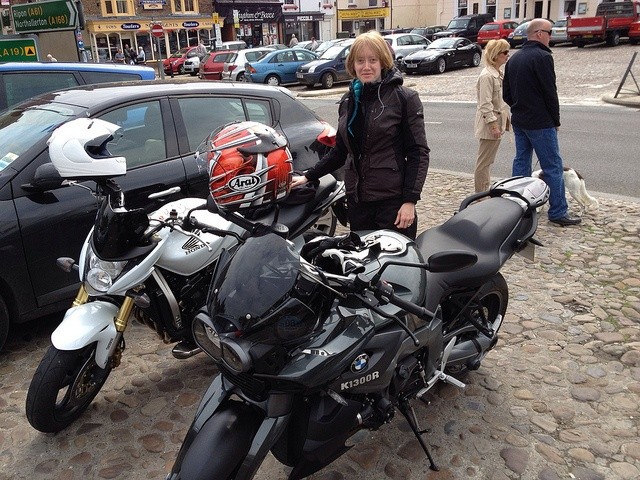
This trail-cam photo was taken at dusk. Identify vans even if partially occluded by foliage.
[182,40,247,77]
[379,27,415,36]
[382,33,432,72]
[409,24,447,40]
[0,63,155,111]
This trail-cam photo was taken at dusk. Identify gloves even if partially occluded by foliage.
[349,241,380,264]
[300,233,363,263]
[311,249,365,277]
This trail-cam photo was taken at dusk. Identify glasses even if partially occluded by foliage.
[535,30,552,35]
[499,51,509,55]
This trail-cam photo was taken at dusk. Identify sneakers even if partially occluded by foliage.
[550,214,581,225]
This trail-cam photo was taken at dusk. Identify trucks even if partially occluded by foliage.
[567,1,640,48]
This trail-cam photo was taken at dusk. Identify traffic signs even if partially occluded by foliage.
[11,1,79,34]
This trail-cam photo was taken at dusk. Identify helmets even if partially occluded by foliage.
[47,118,126,180]
[194,121,293,223]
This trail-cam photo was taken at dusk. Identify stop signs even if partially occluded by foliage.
[151,24,163,38]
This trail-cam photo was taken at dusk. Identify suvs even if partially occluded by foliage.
[432,13,494,42]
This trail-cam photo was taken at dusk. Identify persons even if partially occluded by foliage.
[137,46,144,61]
[291,29,431,241]
[128,49,137,65]
[310,37,319,51]
[502,18,581,224]
[473,39,511,202]
[124,44,130,57]
[46,54,57,62]
[197,39,208,62]
[288,33,298,48]
[115,48,126,63]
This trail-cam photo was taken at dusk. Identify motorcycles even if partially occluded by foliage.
[166,119,552,480]
[25,118,347,432]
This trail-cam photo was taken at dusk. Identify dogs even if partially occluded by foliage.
[531,166,599,217]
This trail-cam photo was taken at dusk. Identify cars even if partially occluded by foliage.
[507,21,531,48]
[292,40,324,52]
[296,38,360,89]
[244,48,320,86]
[628,21,640,45]
[163,46,196,76]
[198,50,236,79]
[400,37,482,76]
[315,38,352,57]
[222,48,277,83]
[477,20,519,49]
[548,19,567,47]
[1,79,348,355]
[255,44,288,49]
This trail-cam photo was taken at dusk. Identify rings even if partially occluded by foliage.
[408,224,411,226]
